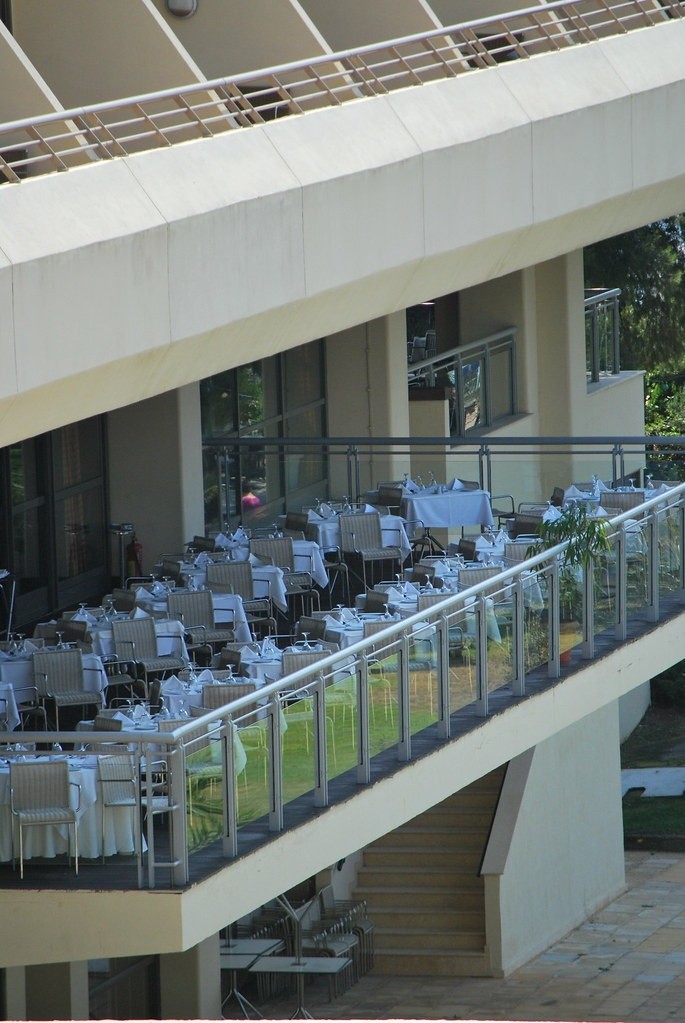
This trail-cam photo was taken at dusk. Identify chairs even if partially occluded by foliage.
[554,478,685,614]
[0,480,553,880]
[234,884,376,1005]
[408,330,436,387]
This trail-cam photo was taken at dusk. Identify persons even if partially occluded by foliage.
[240,484,261,512]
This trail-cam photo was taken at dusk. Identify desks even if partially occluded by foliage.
[451,533,595,576]
[35,612,190,674]
[232,640,362,707]
[1,751,146,872]
[214,537,329,611]
[570,485,670,507]
[278,509,411,609]
[356,588,503,640]
[102,589,252,646]
[1,649,111,728]
[147,669,287,721]
[316,613,446,660]
[543,510,645,554]
[220,885,353,1019]
[364,488,493,557]
[70,715,246,780]
[146,561,286,645]
[404,559,544,614]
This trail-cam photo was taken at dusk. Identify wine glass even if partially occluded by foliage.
[403,468,438,492]
[188,665,197,683]
[343,495,351,515]
[442,550,450,567]
[454,552,464,569]
[251,632,262,653]
[382,604,392,619]
[226,664,236,684]
[264,636,274,655]
[159,696,171,720]
[336,603,345,621]
[126,699,134,720]
[440,578,448,591]
[179,700,189,718]
[7,523,283,653]
[141,700,149,722]
[485,473,654,568]
[302,632,311,650]
[425,574,433,589]
[314,498,324,514]
[395,574,404,591]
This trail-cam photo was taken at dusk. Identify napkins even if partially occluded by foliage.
[0,478,672,763]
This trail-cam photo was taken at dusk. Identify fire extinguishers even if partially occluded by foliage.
[126,531,144,588]
